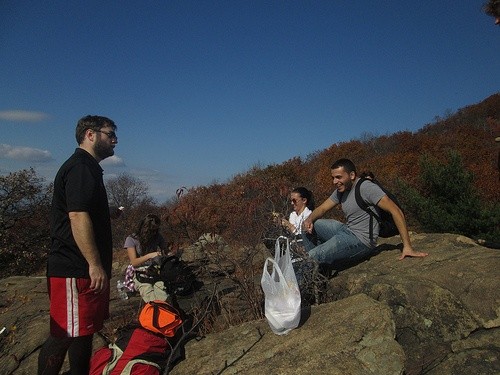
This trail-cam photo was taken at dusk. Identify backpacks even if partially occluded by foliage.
[156,256,196,296]
[339,176,405,238]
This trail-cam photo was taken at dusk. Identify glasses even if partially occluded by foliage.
[289,199,302,204]
[90,128,118,140]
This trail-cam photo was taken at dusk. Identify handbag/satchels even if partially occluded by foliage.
[138,299,182,338]
[261,235,301,336]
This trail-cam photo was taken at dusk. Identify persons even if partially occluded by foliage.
[263,187,316,255]
[124,215,174,306]
[301,158,429,305]
[37,115,118,375]
